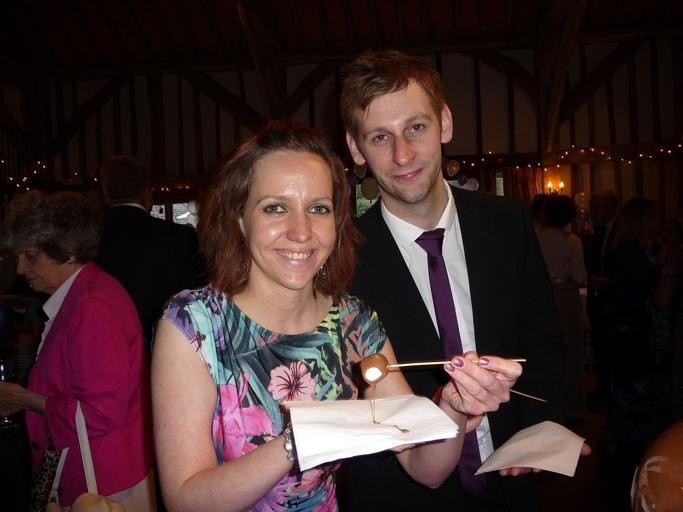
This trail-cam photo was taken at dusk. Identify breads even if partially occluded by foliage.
[359,352,391,387]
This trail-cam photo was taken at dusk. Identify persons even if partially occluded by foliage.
[325,51,596,510]
[529,189,683,455]
[145,115,526,510]
[0,186,155,512]
[89,158,198,352]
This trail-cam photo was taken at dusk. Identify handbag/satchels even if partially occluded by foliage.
[43,401,125,512]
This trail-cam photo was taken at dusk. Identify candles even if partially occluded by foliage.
[75,395,101,498]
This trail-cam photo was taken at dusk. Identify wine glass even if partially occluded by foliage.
[0,359,18,430]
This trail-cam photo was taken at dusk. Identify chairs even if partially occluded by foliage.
[550,274,607,373]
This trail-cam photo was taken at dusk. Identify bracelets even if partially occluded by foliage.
[282,423,299,472]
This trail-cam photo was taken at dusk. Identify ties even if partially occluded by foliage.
[415,227,487,493]
